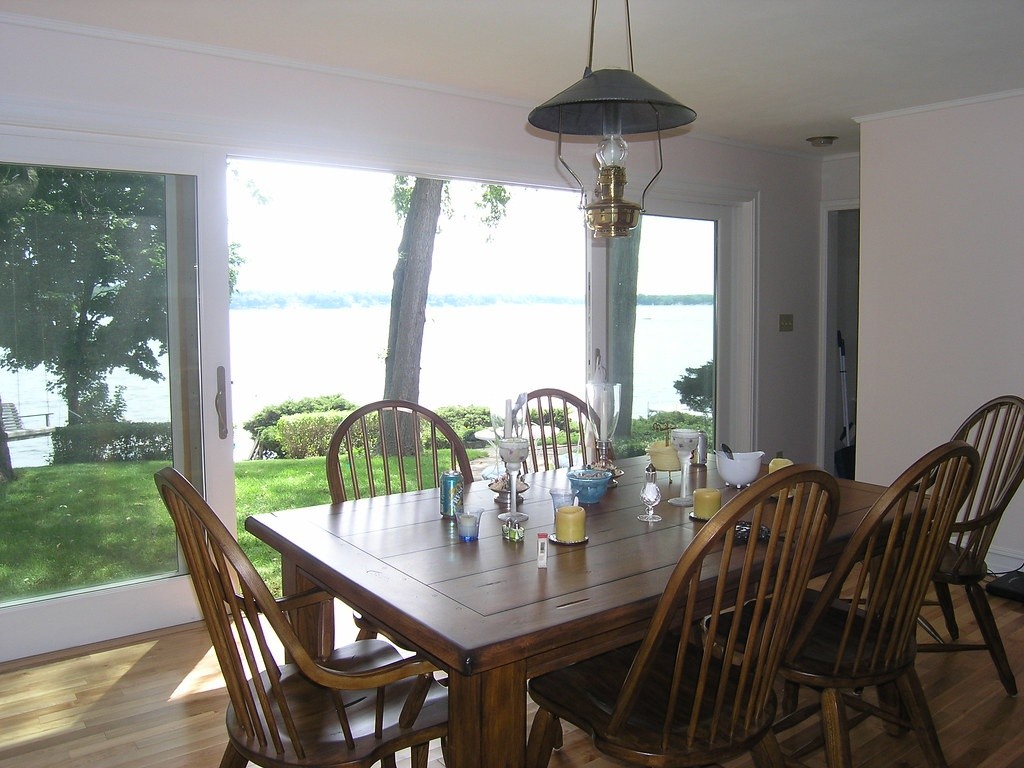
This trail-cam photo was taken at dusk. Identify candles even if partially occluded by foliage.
[555,506,586,541]
[601,388,607,441]
[504,398,513,439]
[693,488,722,518]
[550,488,580,517]
[453,505,485,541]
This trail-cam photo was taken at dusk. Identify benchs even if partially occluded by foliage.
[1,403,60,441]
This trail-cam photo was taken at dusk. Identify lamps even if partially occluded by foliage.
[528,0,696,238]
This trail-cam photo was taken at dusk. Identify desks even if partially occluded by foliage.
[244,450,942,768]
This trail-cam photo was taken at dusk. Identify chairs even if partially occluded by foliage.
[153,388,1024,768]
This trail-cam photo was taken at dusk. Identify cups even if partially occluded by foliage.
[456,508,484,541]
[549,488,579,517]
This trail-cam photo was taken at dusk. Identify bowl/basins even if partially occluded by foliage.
[566,470,612,504]
[714,450,764,488]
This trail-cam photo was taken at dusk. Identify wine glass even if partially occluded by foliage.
[495,438,530,522]
[668,429,699,506]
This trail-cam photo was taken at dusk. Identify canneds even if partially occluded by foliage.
[439,470,464,517]
